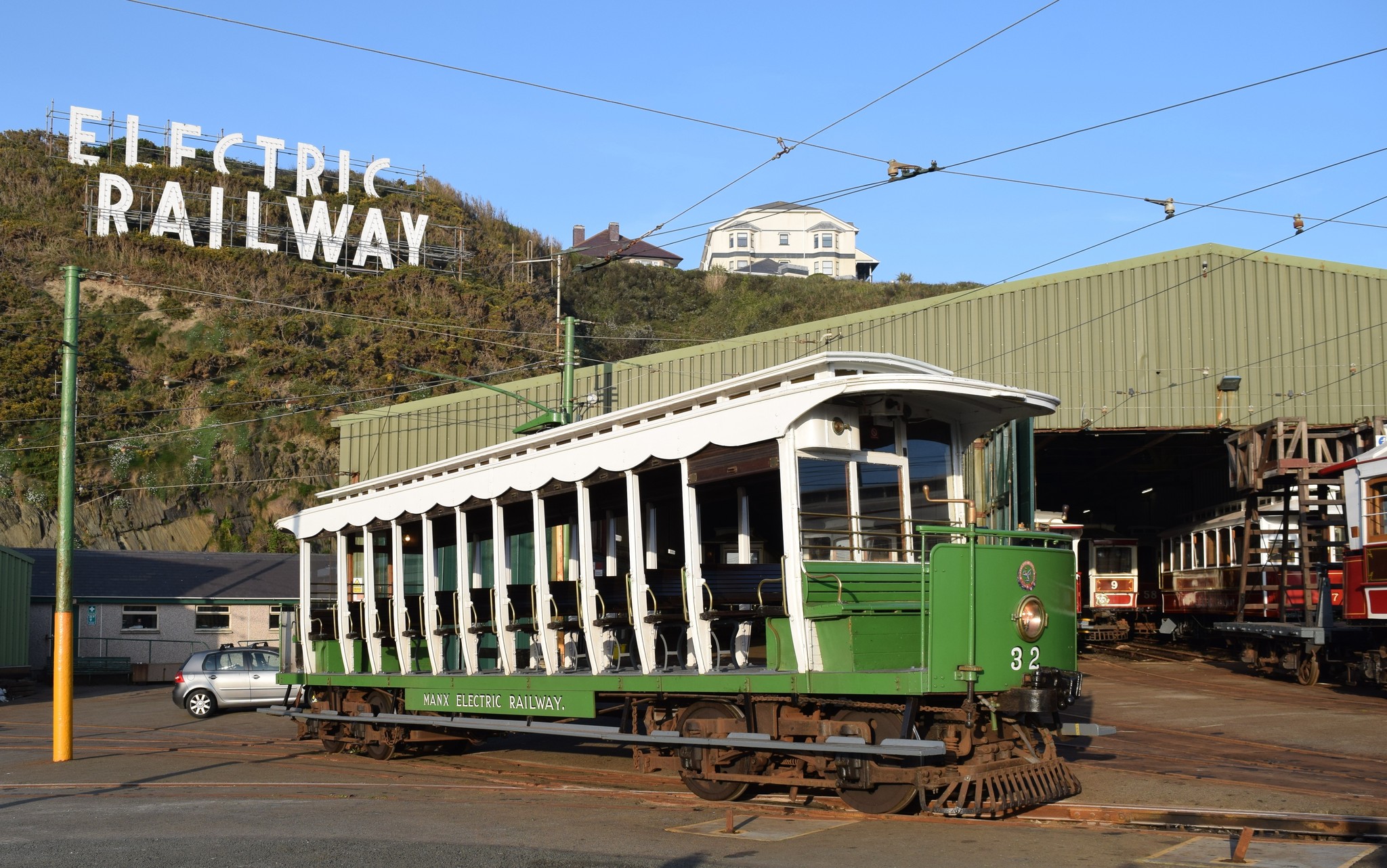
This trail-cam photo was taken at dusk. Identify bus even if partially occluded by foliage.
[1074,536,1139,649]
[1154,437,1387,700]
[252,349,1118,821]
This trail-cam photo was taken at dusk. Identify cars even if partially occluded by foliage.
[171,641,322,719]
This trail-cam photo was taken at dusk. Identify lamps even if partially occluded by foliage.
[1217,375,1242,392]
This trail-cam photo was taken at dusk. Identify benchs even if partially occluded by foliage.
[292,563,931,674]
[47,655,132,686]
